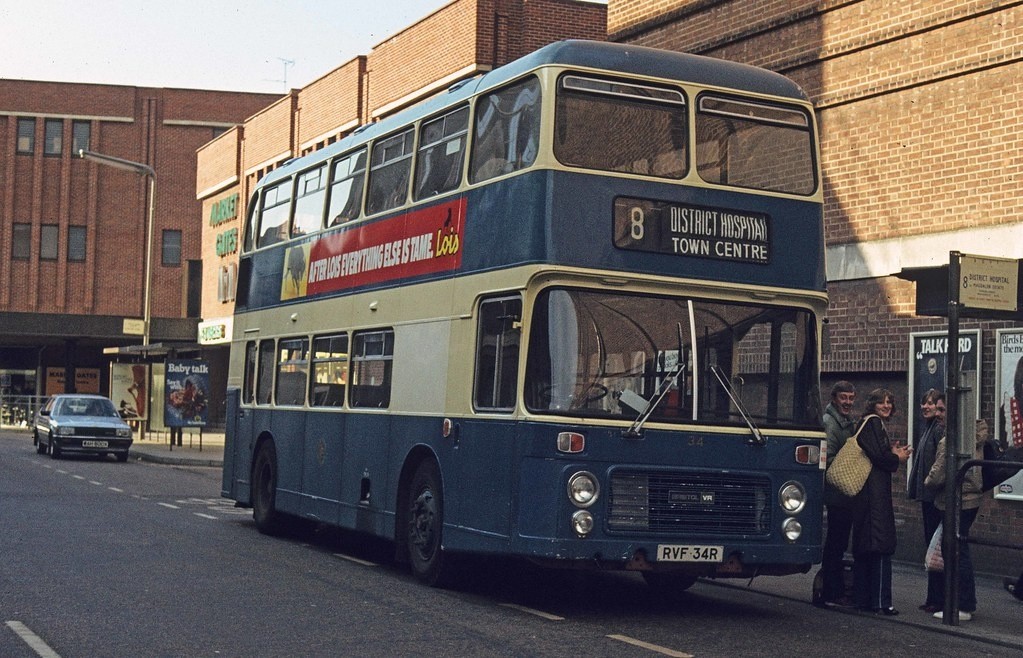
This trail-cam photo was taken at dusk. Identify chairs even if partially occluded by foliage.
[61,407,75,415]
[84,407,94,416]
[253,397,389,408]
[346,189,443,222]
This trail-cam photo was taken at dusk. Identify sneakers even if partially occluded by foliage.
[958,610,971,621]
[933,611,943,618]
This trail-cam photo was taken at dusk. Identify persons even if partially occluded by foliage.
[923,390,989,621]
[906,386,947,611]
[849,388,915,616]
[818,380,859,609]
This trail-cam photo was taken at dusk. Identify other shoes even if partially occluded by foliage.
[873,606,900,615]
[1003,577,1021,600]
[918,605,944,612]
[824,596,859,608]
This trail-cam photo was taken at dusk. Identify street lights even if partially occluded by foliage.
[80,148,159,362]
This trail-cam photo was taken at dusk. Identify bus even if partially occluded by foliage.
[221,38,831,602]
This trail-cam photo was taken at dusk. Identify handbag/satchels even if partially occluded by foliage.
[981,439,1020,493]
[825,435,874,497]
[924,519,945,573]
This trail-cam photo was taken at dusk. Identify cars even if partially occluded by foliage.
[35,394,133,462]
